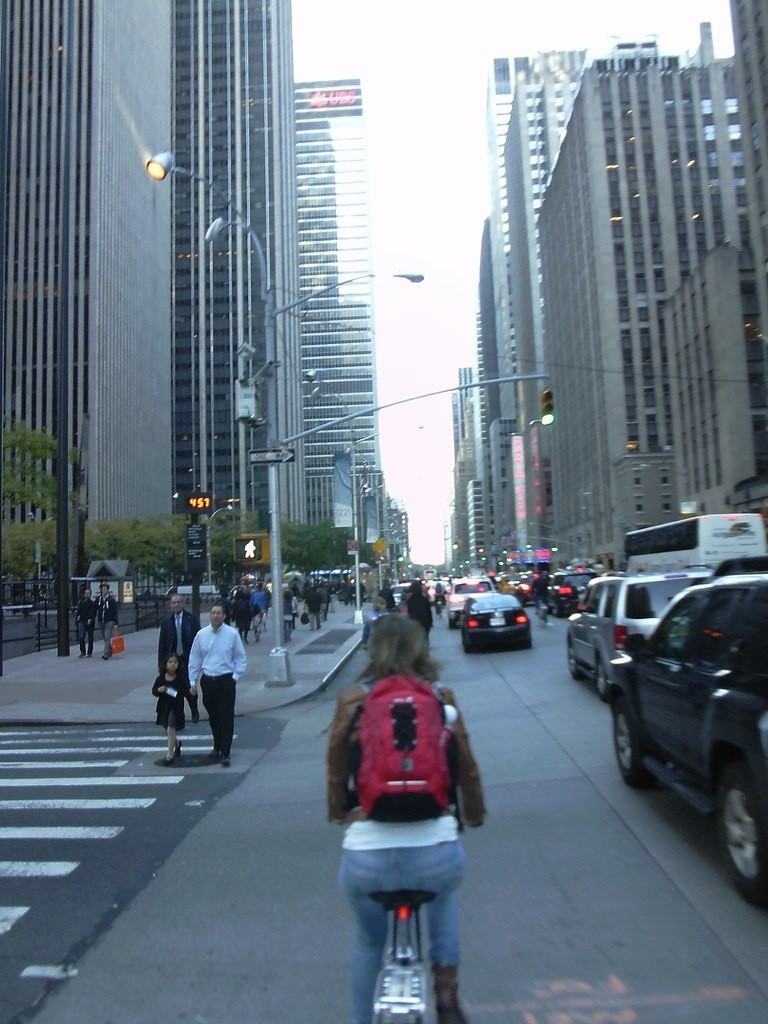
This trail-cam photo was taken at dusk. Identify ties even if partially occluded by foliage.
[176,615,184,656]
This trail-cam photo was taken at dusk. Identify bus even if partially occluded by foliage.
[623,513,767,569]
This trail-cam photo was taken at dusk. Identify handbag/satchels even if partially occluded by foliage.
[110,629,125,654]
[252,605,262,618]
[301,612,309,625]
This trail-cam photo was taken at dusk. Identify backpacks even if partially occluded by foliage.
[342,674,464,835]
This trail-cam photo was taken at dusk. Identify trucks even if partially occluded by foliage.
[165,585,216,594]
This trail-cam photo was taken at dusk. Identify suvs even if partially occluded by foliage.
[607,555,768,910]
[566,564,716,703]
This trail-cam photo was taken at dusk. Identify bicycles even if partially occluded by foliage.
[536,600,548,626]
[369,889,438,1024]
[435,592,443,619]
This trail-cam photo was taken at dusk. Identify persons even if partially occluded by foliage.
[74,589,97,657]
[187,604,247,767]
[152,653,199,767]
[327,615,487,1024]
[532,571,552,620]
[362,579,447,651]
[158,595,201,724]
[216,574,366,644]
[95,584,118,660]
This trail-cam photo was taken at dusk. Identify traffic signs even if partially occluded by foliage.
[249,446,296,463]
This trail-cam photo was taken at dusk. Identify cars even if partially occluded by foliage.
[390,567,602,652]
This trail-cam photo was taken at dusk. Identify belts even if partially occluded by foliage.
[204,674,230,680]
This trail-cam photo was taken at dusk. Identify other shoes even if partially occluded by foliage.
[432,983,466,1023]
[164,757,174,764]
[174,742,183,758]
[101,656,109,660]
[210,749,218,757]
[79,653,86,657]
[221,755,230,766]
[192,711,199,723]
[88,653,92,657]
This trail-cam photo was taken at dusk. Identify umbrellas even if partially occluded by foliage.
[284,571,302,581]
[241,574,258,582]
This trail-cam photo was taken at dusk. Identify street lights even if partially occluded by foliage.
[302,370,424,625]
[147,150,425,682]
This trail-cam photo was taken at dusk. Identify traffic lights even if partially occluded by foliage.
[539,389,555,427]
[233,534,270,564]
